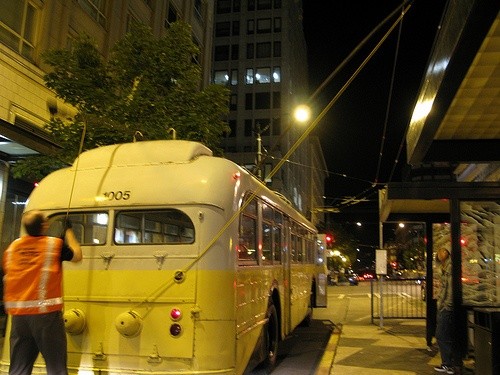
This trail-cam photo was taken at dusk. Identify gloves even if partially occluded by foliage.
[63,216,71,228]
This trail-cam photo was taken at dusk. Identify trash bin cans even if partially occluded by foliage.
[473,308,500,375]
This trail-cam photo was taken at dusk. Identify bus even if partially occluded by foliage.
[2,139,327,375]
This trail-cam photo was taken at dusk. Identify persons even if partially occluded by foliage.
[2,210,83,375]
[434,248,455,374]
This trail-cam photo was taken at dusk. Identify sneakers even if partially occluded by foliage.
[454,359,463,369]
[433,362,455,374]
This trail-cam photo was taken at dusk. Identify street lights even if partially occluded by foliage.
[255,104,311,180]
